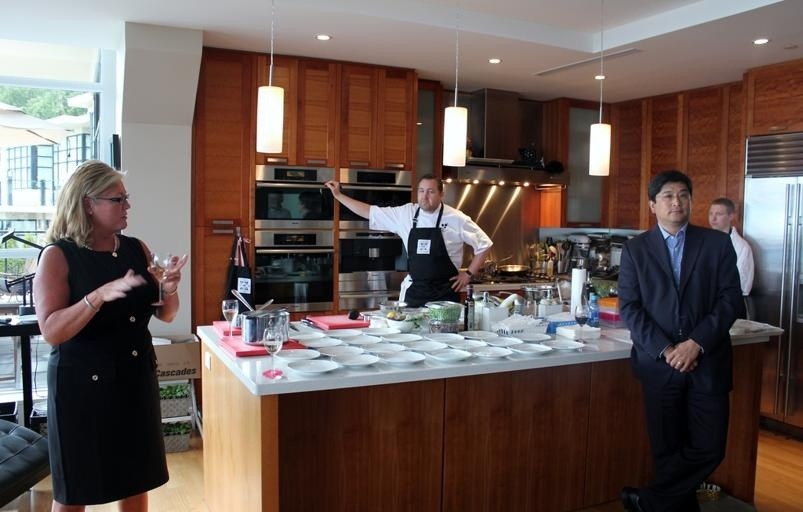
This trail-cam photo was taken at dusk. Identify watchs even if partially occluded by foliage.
[465,270,475,280]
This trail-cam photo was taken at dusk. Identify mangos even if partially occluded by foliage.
[387,310,396,318]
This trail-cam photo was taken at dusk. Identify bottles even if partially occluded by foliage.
[588,295,598,327]
[463,285,474,330]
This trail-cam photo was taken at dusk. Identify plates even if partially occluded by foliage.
[513,344,552,355]
[364,330,400,335]
[404,342,447,351]
[473,348,513,359]
[327,331,362,337]
[541,340,581,351]
[382,352,426,365]
[384,333,422,343]
[287,331,325,338]
[514,333,551,342]
[276,349,319,361]
[449,340,487,349]
[423,333,464,344]
[342,335,382,344]
[365,344,406,354]
[332,355,380,366]
[461,332,498,339]
[301,337,343,349]
[486,337,522,348]
[319,346,364,357]
[425,349,471,360]
[287,359,340,374]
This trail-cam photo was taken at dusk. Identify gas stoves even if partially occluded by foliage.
[468,274,551,284]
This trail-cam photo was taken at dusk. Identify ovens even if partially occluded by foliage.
[254,164,413,308]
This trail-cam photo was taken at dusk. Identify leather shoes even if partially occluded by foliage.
[621,487,642,512]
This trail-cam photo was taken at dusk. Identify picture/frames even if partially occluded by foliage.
[107,134,120,171]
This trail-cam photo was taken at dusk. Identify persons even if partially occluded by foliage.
[298,189,325,220]
[322,172,494,307]
[618,170,742,512]
[704,197,755,321]
[269,190,292,218]
[33,158,189,511]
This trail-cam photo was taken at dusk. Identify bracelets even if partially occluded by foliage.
[83,294,101,313]
[163,286,179,297]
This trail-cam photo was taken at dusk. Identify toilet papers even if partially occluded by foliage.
[571,267,586,315]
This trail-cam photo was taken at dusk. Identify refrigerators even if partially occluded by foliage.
[742,178,802,434]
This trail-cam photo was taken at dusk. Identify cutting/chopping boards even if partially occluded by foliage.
[306,314,369,331]
[219,337,304,357]
[212,320,242,336]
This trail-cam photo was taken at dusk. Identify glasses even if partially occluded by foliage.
[85,194,129,205]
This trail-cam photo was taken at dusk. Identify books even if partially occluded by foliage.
[211,319,243,336]
[217,333,306,358]
[305,313,374,330]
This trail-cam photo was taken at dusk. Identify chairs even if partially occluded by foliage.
[1,419,51,509]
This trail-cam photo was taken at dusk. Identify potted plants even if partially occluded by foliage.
[162,421,192,453]
[160,384,189,417]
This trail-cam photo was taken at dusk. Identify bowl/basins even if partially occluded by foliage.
[377,301,408,313]
[385,315,416,333]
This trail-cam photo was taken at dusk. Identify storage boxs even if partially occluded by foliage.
[151,334,201,381]
[598,297,626,329]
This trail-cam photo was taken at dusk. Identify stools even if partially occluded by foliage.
[0,313,42,429]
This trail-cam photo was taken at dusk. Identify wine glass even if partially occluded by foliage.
[574,305,589,352]
[222,300,239,344]
[149,252,173,308]
[264,326,282,380]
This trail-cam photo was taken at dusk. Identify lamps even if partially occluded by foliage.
[254,0,285,155]
[443,27,468,168]
[589,0,612,177]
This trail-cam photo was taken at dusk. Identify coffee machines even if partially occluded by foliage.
[567,235,589,274]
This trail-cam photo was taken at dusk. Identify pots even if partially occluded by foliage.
[241,309,291,345]
[497,265,531,276]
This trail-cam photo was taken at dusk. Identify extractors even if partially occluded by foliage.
[439,89,571,191]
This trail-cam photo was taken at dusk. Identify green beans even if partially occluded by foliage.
[158,382,191,399]
[159,422,193,435]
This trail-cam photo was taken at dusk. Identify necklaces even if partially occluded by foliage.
[76,231,118,254]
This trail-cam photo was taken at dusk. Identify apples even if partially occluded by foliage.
[347,308,360,320]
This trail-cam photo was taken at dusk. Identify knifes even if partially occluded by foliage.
[301,320,325,331]
[289,323,299,332]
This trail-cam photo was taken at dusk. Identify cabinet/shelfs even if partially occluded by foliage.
[335,60,417,168]
[190,44,256,333]
[256,51,335,167]
[161,379,196,450]
